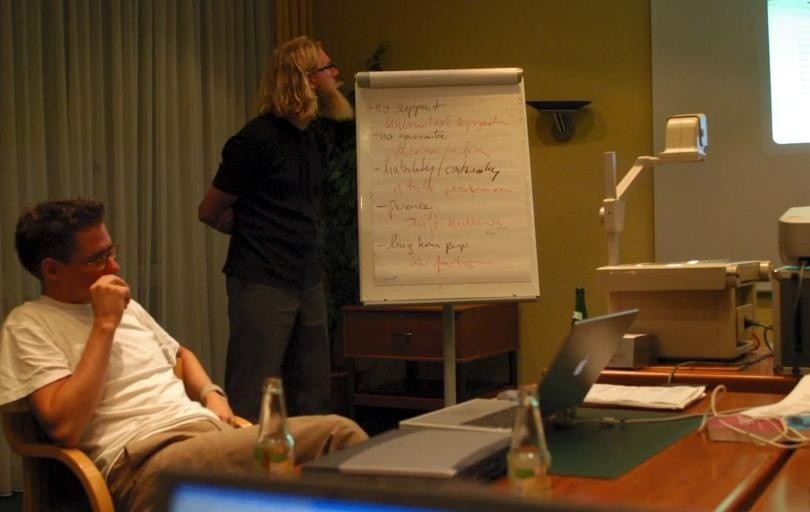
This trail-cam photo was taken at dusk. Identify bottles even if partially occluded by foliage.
[569,287,588,328]
[507,381,553,501]
[254,376,298,475]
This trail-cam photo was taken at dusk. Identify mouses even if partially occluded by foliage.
[497,390,519,401]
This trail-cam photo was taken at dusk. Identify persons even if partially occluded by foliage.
[0,197,372,512]
[197,34,356,425]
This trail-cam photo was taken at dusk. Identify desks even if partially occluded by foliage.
[543,346,809,394]
[295,381,809,512]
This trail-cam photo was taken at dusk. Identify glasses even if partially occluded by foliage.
[318,58,336,76]
[70,241,118,271]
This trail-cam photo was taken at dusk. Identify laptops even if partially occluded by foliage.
[399,308,639,440]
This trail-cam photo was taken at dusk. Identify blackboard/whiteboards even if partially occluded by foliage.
[355,69,540,307]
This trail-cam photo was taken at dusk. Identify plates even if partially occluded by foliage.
[524,100,592,109]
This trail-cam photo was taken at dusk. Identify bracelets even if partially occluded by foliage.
[199,382,229,406]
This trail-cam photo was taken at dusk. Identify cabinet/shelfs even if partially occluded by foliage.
[344,304,524,430]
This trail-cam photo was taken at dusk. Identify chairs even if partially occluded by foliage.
[3,412,254,512]
